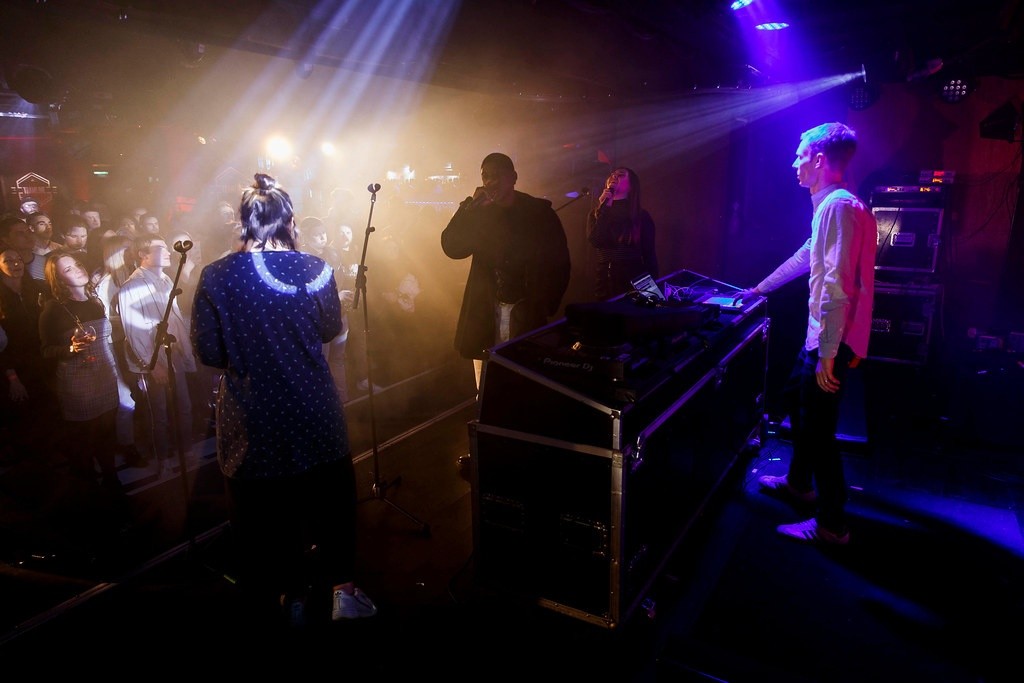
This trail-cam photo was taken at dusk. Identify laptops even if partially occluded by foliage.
[631,273,667,302]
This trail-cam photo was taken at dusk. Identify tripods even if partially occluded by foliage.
[349,183,432,531]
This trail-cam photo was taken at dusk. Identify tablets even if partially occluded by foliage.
[702,297,750,309]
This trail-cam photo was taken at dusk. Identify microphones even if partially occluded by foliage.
[467,184,497,209]
[600,187,615,210]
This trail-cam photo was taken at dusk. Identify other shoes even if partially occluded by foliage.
[456,453,473,473]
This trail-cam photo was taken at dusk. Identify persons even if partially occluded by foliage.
[588,168,657,295]
[187,174,377,628]
[441,154,571,486]
[286,162,417,391]
[722,122,879,543]
[0,194,244,477]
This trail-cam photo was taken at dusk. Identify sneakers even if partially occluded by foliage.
[758,473,816,501]
[776,517,850,546]
[332,581,377,621]
